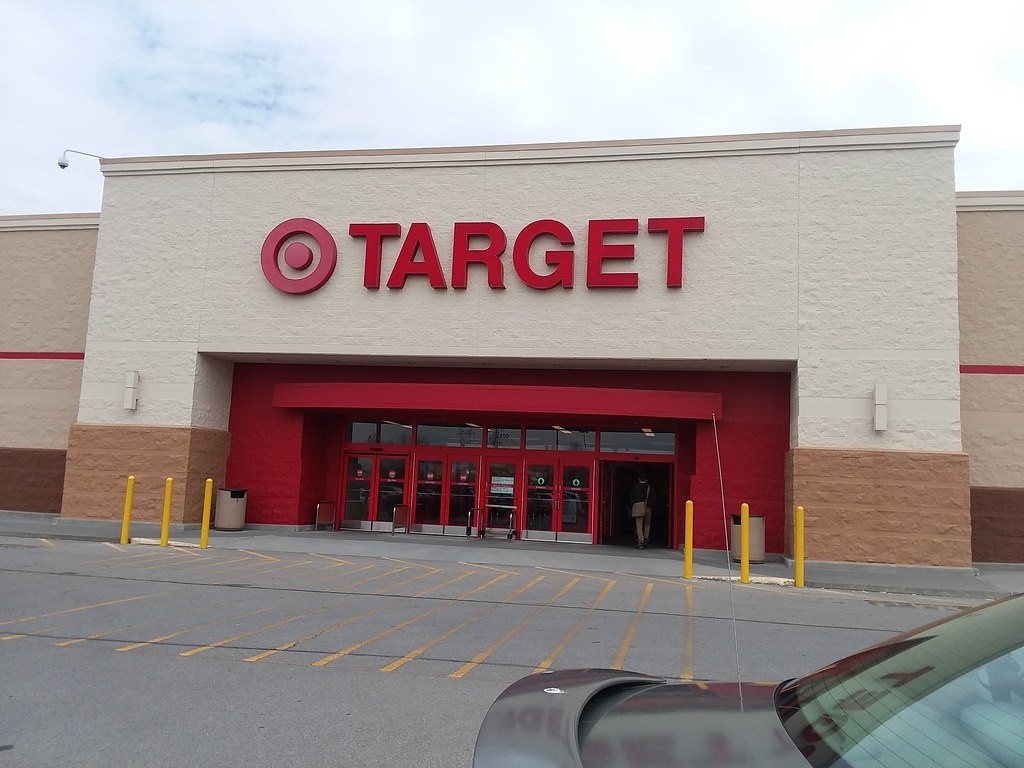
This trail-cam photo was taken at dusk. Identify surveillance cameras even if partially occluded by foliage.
[58,158,68,169]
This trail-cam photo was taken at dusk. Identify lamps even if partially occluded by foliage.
[123,370,139,411]
[874,382,888,432]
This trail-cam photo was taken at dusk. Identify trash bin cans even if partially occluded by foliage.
[730,513,766,564]
[213,487,250,531]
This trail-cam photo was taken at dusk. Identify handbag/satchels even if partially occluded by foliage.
[631,502,646,517]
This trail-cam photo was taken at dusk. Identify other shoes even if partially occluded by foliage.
[644,543,648,548]
[638,545,644,549]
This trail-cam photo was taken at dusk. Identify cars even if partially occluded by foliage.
[470,591,1024,768]
[368,483,588,517]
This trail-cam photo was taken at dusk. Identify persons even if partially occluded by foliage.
[628,473,657,549]
